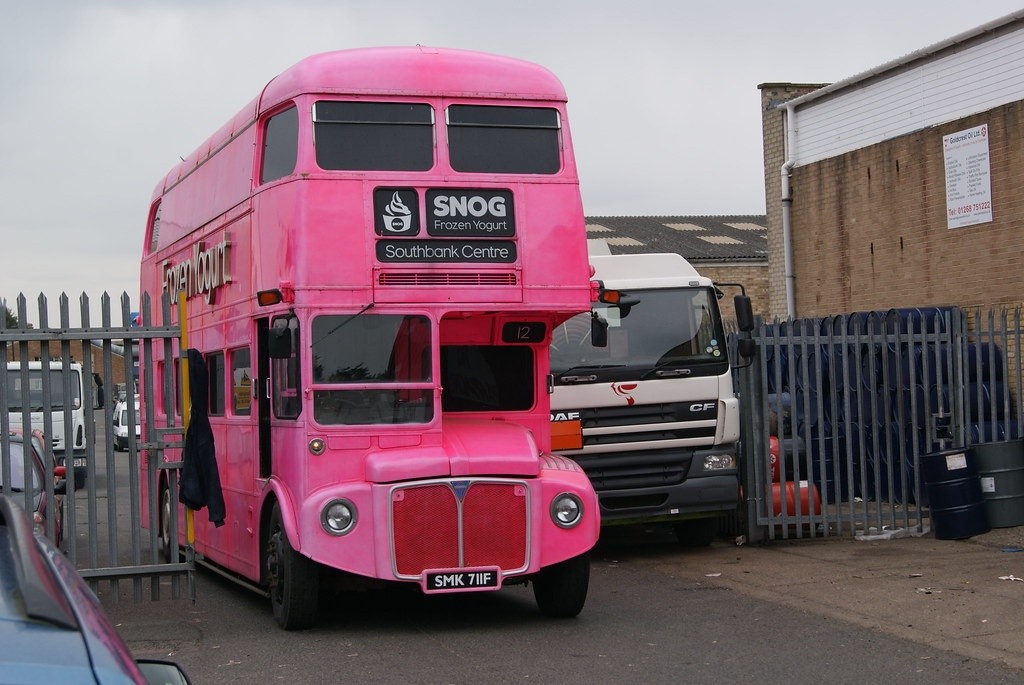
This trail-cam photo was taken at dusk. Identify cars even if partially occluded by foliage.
[0,494,191,684]
[0,433,77,548]
[9,428,67,540]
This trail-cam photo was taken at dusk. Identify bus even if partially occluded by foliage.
[139,43,610,632]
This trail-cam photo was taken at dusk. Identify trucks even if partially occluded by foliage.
[6,357,105,489]
[549,240,757,553]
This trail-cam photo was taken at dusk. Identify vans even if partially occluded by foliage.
[113,398,141,452]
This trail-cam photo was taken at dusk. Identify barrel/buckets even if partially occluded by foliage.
[969,438,1024,528]
[919,446,991,540]
[727,307,1015,530]
